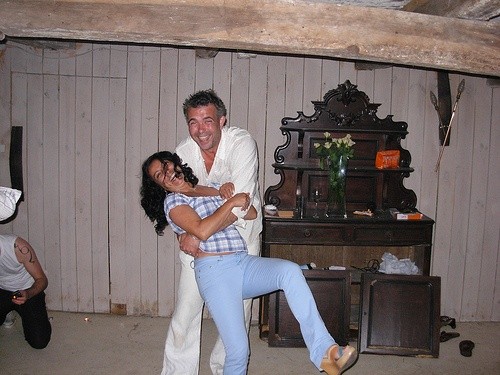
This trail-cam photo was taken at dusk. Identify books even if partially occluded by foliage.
[276,208,293,218]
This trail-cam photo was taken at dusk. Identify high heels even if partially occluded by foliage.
[321,344,358,375]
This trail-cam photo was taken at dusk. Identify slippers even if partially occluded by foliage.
[460,340,474,357]
[439,331,459,342]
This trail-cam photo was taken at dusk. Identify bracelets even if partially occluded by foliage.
[190,233,202,241]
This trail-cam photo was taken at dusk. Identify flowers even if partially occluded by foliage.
[313,131,356,204]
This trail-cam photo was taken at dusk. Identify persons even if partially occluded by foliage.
[0,234,53,350]
[139,151,359,375]
[159,89,263,375]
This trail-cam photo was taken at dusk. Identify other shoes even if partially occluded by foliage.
[2,310,17,328]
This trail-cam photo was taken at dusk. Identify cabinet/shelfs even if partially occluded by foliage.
[258,79,442,358]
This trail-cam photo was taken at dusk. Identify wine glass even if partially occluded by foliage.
[312,186,322,218]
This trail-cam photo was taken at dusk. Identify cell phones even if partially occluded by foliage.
[9,291,22,299]
